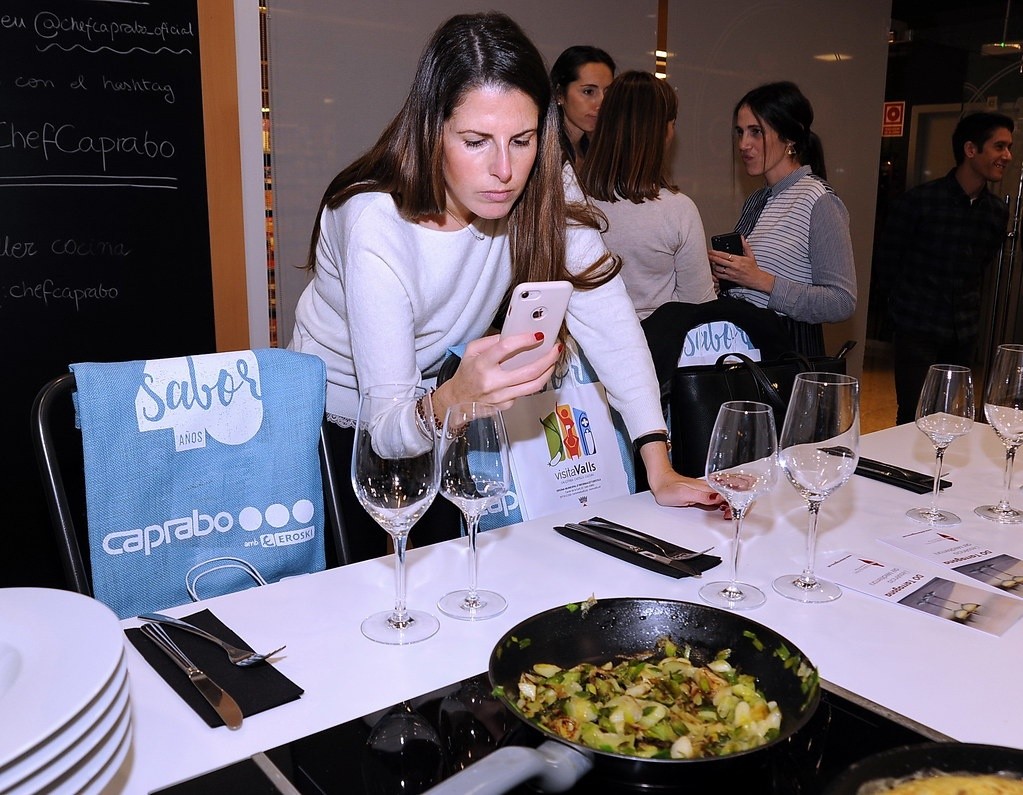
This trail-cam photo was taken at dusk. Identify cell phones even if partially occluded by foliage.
[711,232,746,291]
[499,281,574,370]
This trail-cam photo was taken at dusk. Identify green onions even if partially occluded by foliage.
[493,605,815,759]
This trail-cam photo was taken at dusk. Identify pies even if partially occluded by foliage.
[869,775,1023,795]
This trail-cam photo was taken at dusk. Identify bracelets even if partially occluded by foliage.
[417,388,468,437]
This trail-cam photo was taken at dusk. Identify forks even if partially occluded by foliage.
[137,612,286,665]
[859,458,949,482]
[579,520,715,560]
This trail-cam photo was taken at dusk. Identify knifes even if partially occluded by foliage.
[564,523,703,579]
[857,464,944,492]
[140,623,243,730]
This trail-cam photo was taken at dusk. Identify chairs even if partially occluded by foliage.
[31,348,349,596]
[437,330,638,541]
[610,297,797,493]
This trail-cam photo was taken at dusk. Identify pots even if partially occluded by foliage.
[425,597,820,795]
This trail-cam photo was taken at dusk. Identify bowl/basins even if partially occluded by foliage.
[828,744,1023,795]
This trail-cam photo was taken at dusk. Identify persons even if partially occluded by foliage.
[286,11,755,566]
[493,45,616,331]
[708,80,857,358]
[580,70,719,320]
[873,111,1015,425]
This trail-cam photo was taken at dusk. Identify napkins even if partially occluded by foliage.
[818,446,954,495]
[552,515,722,579]
[123,607,305,728]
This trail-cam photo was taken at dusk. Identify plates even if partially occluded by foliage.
[1,588,133,794]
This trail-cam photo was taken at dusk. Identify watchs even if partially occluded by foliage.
[633,431,672,454]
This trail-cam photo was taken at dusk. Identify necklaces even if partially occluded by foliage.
[445,207,488,241]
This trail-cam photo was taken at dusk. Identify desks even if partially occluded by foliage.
[95,411,1023,795]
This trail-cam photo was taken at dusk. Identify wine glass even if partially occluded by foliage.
[771,372,860,603]
[916,591,987,623]
[349,384,443,645]
[970,563,1023,592]
[974,344,1023,525]
[906,365,975,526]
[434,402,511,621]
[698,400,779,611]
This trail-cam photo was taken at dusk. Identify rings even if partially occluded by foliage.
[723,267,726,273]
[727,254,732,260]
